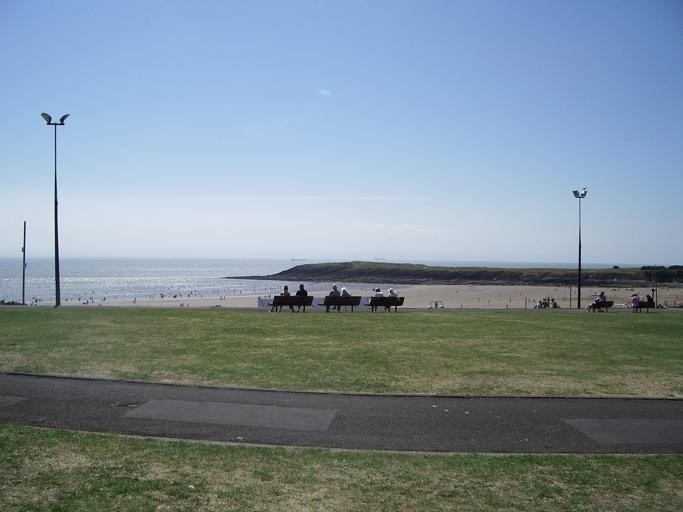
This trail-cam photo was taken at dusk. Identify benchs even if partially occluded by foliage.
[318,295,361,312]
[267,296,314,312]
[364,297,404,313]
[632,302,655,312]
[588,301,614,312]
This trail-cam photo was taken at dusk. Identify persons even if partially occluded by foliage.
[279,283,307,312]
[587,292,606,313]
[365,288,394,312]
[535,297,559,309]
[329,283,351,313]
[630,294,653,313]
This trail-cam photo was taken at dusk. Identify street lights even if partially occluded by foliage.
[38,112,67,307]
[572,188,589,309]
